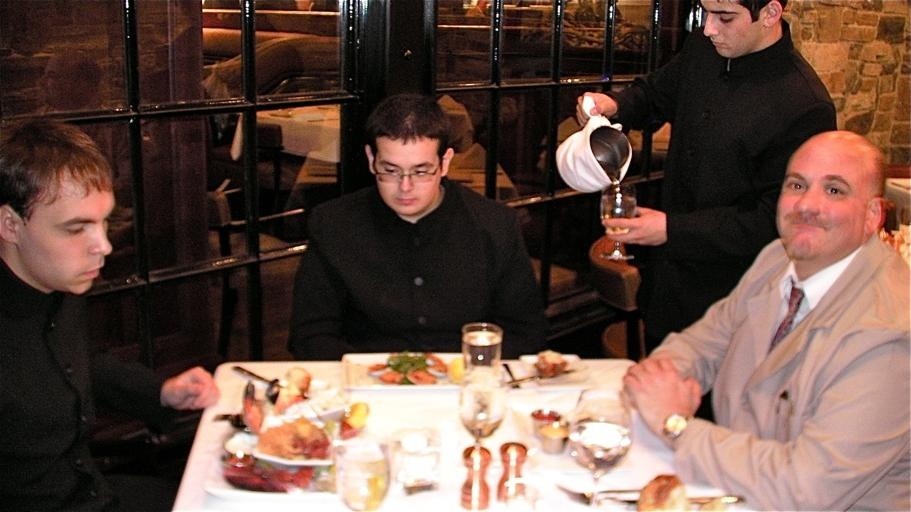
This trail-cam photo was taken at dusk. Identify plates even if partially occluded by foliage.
[338,350,476,395]
[515,351,592,387]
[223,415,346,468]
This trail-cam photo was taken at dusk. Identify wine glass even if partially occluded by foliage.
[564,383,638,511]
[329,433,394,512]
[454,378,512,465]
[596,182,638,262]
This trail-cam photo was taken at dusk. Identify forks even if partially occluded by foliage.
[553,483,652,506]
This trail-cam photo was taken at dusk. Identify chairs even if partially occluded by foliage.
[204,188,308,362]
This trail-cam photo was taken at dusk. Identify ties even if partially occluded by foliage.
[771,288,804,351]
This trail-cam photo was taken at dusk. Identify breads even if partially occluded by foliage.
[448,356,464,380]
[636,473,689,512]
[534,349,569,376]
[341,402,369,430]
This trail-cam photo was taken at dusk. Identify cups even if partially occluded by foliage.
[458,320,509,391]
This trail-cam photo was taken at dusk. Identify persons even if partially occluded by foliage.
[0,118,220,510]
[623,132,911,510]
[32,48,130,212]
[576,0,838,359]
[287,92,540,359]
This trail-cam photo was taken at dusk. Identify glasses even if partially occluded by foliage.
[373,164,439,183]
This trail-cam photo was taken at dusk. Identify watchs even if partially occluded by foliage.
[662,412,691,443]
